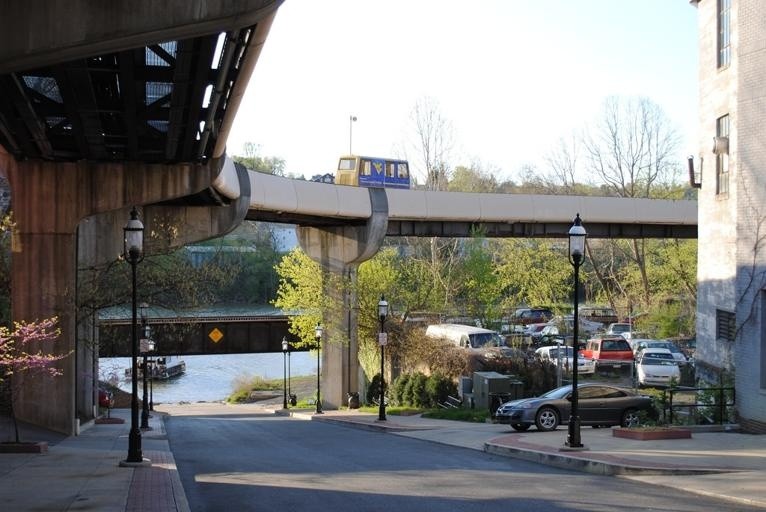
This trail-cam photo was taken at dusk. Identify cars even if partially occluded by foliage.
[399,304,689,431]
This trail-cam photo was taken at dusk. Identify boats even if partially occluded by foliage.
[125,355,186,380]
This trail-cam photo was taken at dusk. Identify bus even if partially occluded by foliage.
[335,155,410,189]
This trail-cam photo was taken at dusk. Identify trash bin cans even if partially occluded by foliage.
[347,392,359,409]
[488,392,511,420]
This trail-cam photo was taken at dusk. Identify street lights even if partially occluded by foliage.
[560,213,591,450]
[119,203,156,468]
[378,294,388,421]
[315,321,323,413]
[349,116,357,156]
[281,336,289,409]
